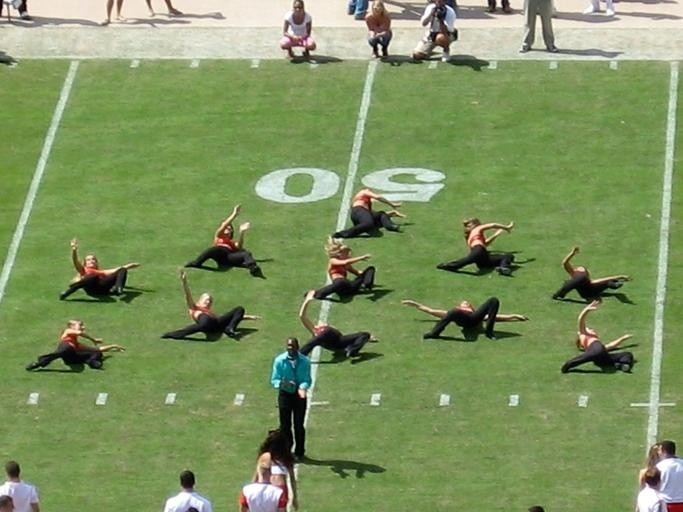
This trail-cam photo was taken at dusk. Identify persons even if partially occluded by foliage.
[0,460,39,512]
[163,430,298,512]
[184,203,262,277]
[298,290,378,358]
[160,264,263,340]
[25,320,125,371]
[303,235,375,298]
[638,440,683,512]
[529,505,543,511]
[271,337,312,463]
[332,189,407,237]
[401,217,634,372]
[59,238,141,301]
